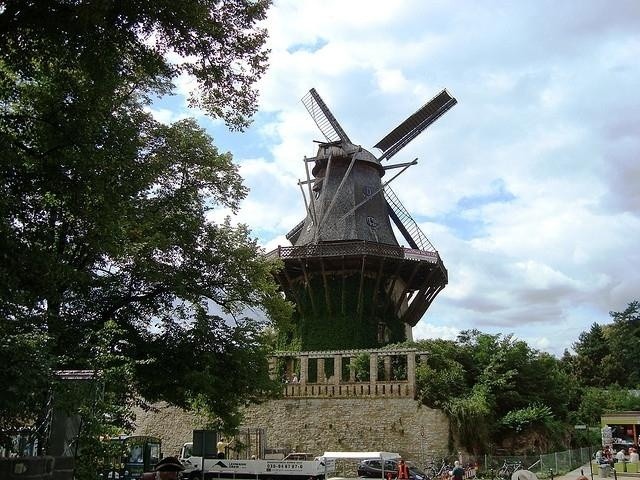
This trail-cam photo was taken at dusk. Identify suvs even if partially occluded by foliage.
[358,458,430,480]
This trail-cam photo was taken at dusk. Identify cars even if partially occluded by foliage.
[140,463,185,480]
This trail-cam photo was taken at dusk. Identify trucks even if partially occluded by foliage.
[177,441,334,480]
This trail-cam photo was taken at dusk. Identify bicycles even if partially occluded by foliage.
[424,458,475,479]
[495,460,525,480]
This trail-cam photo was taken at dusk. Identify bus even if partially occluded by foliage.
[0,429,162,480]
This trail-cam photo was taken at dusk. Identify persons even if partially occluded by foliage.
[448,460,466,480]
[291,373,299,384]
[386,472,393,480]
[396,460,410,480]
[629,448,639,462]
[216,436,234,459]
[595,445,615,468]
[282,371,291,383]
[616,447,627,462]
[440,460,479,480]
